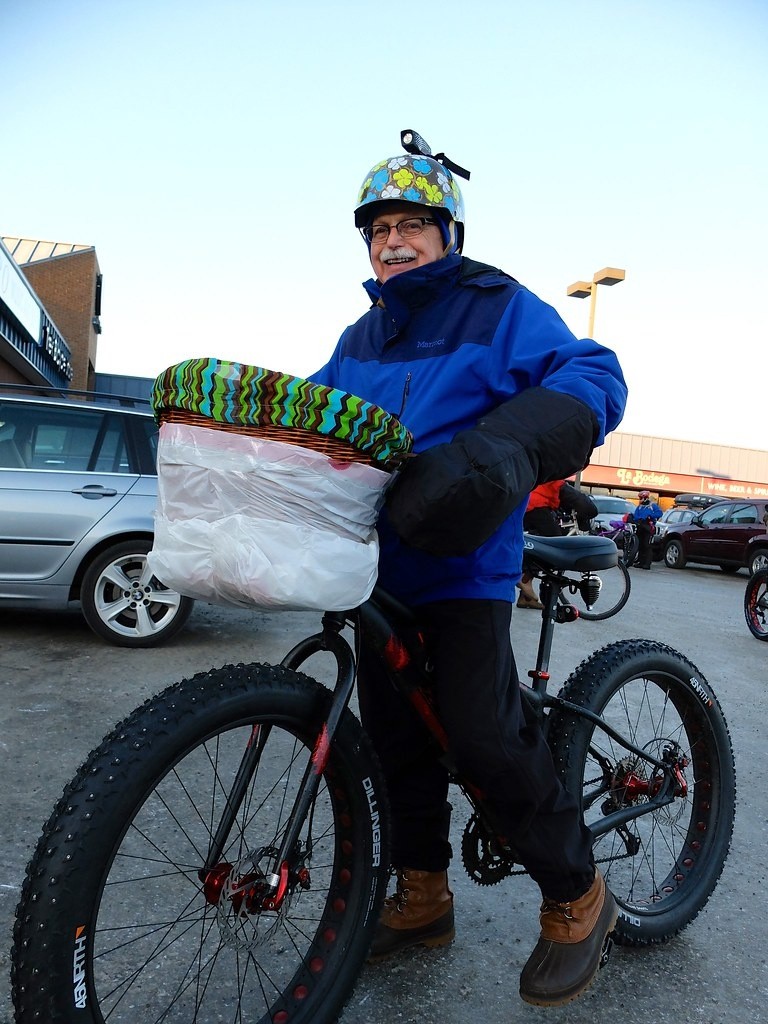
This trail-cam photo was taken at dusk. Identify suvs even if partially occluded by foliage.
[660,497,768,583]
[0,381,196,648]
[651,492,734,561]
[587,492,638,550]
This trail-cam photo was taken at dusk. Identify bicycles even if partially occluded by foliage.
[10,534,741,1023]
[593,518,638,568]
[518,494,631,621]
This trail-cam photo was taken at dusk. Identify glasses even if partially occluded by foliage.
[365,220,441,244]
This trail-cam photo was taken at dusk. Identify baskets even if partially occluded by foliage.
[147,355,412,613]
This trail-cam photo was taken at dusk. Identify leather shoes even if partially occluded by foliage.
[365,869,456,964]
[519,864,620,1007]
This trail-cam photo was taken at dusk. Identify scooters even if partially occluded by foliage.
[742,504,768,643]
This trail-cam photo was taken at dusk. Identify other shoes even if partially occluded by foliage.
[517,588,546,610]
[516,572,539,602]
[634,562,644,568]
[642,564,651,570]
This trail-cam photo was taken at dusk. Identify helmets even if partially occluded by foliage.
[352,153,465,227]
[637,491,651,499]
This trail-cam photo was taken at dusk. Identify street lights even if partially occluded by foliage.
[565,265,625,491]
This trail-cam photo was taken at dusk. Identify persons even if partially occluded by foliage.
[516,480,600,609]
[298,150,620,1007]
[622,490,663,570]
